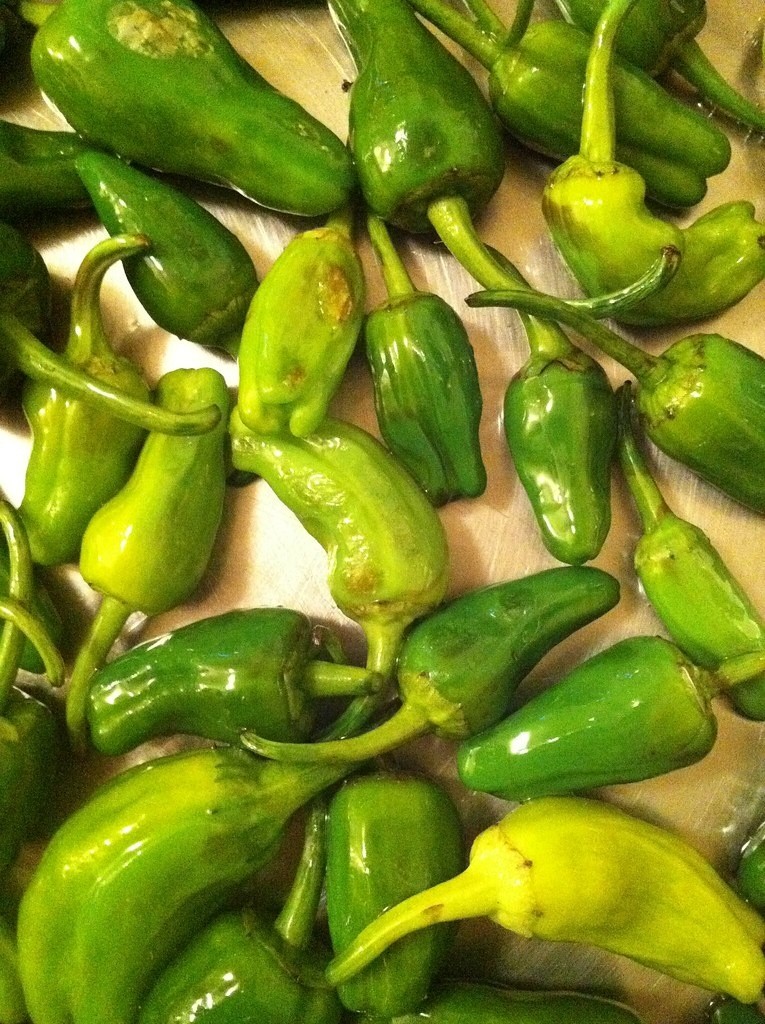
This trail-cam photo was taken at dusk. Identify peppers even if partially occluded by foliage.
[0,0,765,1024]
